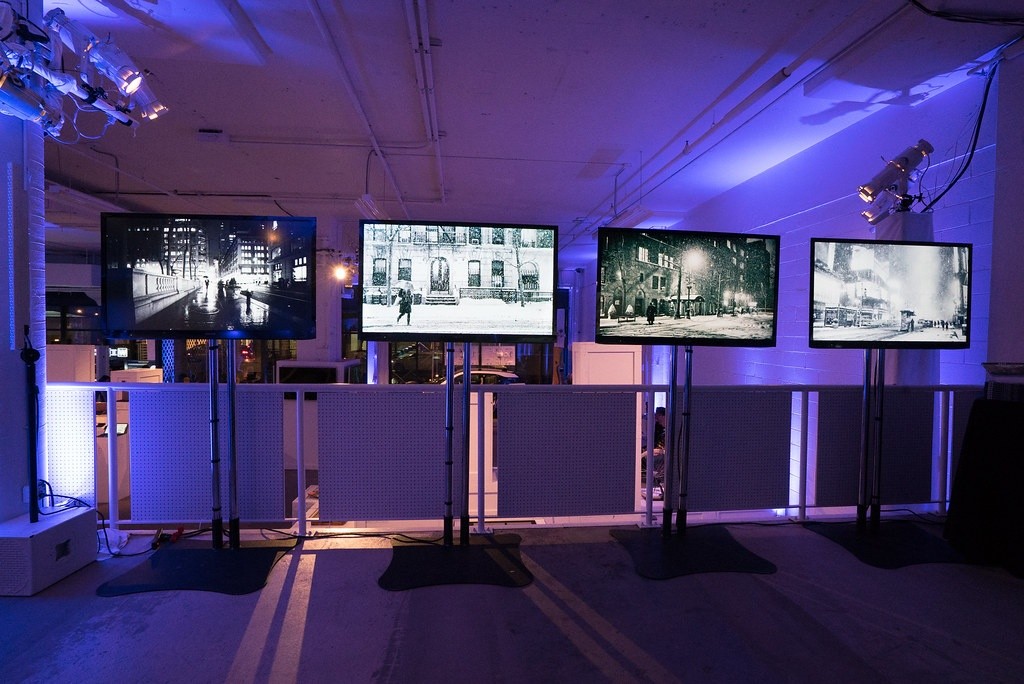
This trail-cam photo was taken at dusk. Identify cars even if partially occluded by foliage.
[436,370,526,384]
[187,343,255,361]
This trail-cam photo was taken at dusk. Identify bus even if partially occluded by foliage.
[824,307,860,328]
[860,308,888,327]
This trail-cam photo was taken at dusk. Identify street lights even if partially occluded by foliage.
[686,260,696,318]
[712,266,725,317]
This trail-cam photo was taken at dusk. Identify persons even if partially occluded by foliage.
[646,303,657,325]
[504,289,518,304]
[397,288,412,326]
[205,277,209,289]
[934,320,949,331]
[863,287,868,298]
[246,288,253,315]
[910,318,915,331]
[655,407,666,450]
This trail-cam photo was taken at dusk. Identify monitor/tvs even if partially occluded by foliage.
[596,227,781,347]
[358,218,560,344]
[100,211,318,339]
[808,237,973,350]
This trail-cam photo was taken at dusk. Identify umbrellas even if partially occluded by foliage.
[391,281,415,291]
[903,317,910,324]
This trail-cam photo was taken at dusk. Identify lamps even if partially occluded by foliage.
[41,7,169,120]
[857,139,934,226]
